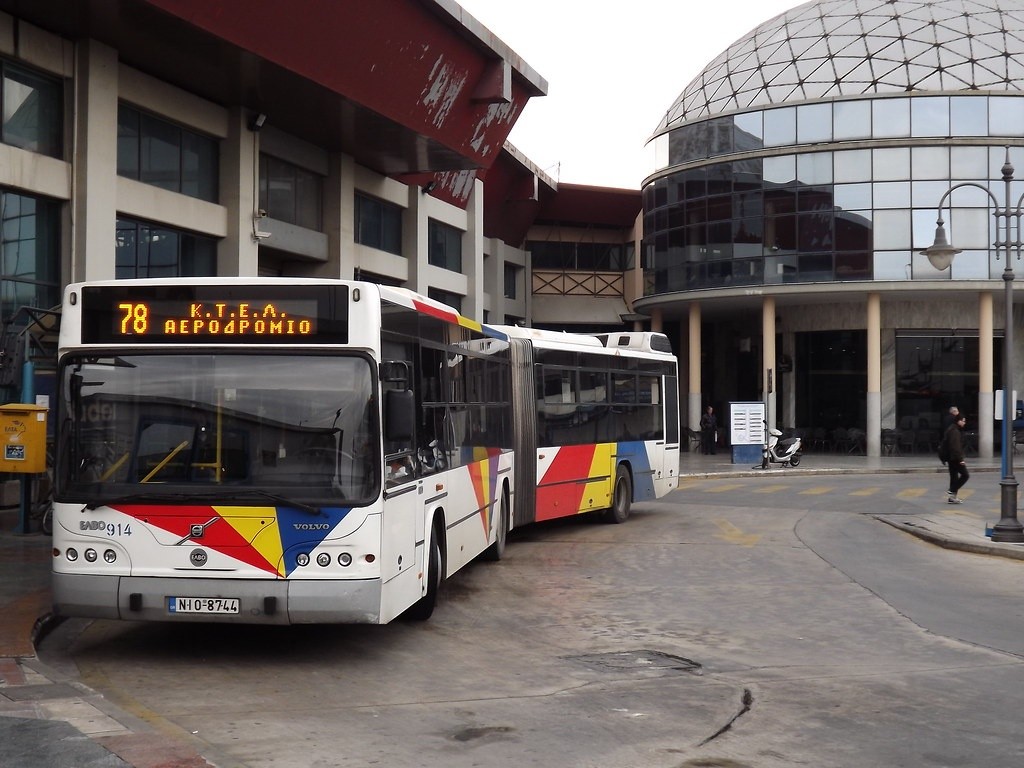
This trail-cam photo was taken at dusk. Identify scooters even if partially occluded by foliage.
[761,420,803,470]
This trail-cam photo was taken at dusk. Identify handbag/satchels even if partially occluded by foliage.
[937,440,949,465]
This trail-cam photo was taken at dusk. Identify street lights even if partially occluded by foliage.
[919,143,1024,544]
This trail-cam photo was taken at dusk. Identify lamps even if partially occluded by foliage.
[423,180,438,193]
[250,114,267,132]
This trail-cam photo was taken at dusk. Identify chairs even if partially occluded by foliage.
[686,426,702,452]
[779,415,981,458]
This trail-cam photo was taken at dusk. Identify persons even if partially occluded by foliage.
[700,406,717,455]
[939,407,971,503]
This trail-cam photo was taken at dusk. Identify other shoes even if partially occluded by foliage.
[945,490,963,505]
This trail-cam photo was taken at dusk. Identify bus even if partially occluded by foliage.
[52,276,683,624]
